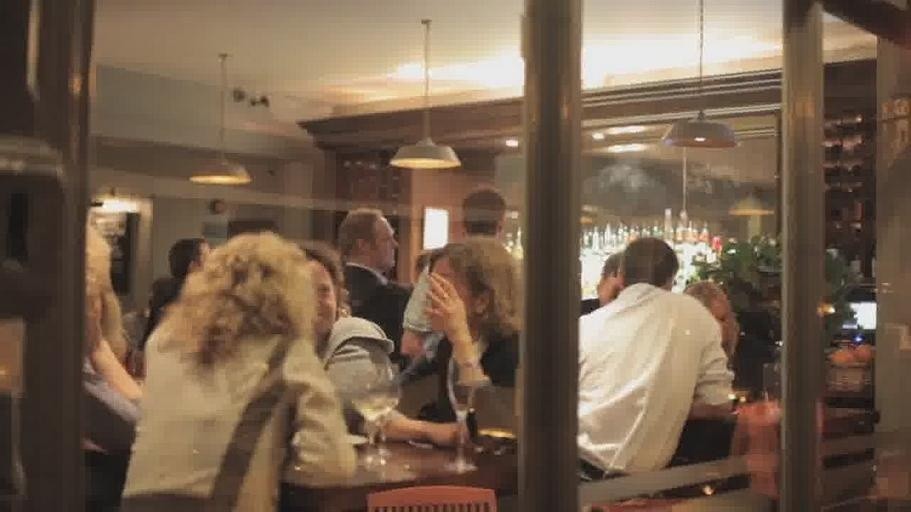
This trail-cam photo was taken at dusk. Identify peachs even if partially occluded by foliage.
[833,344,871,363]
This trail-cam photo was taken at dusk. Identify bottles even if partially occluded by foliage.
[502,217,722,299]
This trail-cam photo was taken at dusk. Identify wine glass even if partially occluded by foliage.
[445,356,479,472]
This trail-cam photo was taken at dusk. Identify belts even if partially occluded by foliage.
[580,457,612,483]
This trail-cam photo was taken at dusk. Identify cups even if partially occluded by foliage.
[347,358,404,473]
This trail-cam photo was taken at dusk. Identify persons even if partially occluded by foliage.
[576,238,782,498]
[75,190,525,511]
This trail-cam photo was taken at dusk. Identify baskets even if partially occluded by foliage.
[825,347,873,399]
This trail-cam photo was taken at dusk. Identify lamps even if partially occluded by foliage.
[390,18,464,171]
[190,52,254,184]
[661,0,739,149]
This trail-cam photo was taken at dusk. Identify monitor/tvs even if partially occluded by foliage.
[842,300,878,331]
